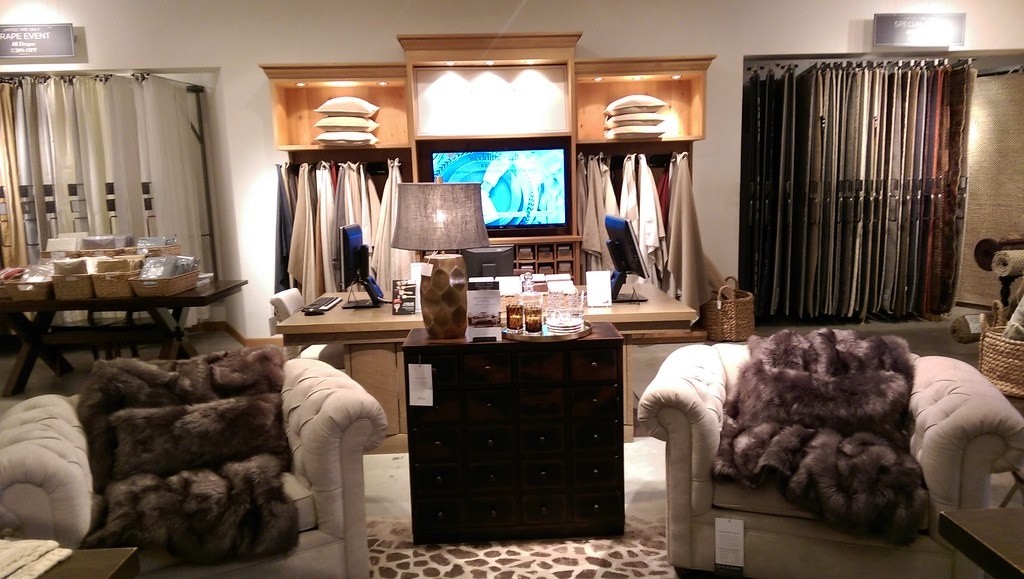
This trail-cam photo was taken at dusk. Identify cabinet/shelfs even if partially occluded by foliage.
[275,283,708,455]
[260,28,718,284]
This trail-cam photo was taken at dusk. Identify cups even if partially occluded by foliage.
[524,292,543,336]
[505,292,523,334]
[546,286,584,333]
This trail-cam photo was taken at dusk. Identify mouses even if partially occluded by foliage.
[304,309,324,316]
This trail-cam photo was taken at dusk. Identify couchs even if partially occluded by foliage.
[634,341,1024,579]
[0,358,387,579]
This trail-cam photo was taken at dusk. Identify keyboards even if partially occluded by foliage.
[302,297,343,311]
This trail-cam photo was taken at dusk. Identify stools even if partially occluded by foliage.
[50,314,138,360]
[106,313,169,359]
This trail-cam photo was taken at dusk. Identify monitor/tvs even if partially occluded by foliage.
[604,215,649,303]
[463,244,513,277]
[431,146,569,236]
[338,224,384,308]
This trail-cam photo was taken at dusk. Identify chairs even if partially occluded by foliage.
[269,286,345,372]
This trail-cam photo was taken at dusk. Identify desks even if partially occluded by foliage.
[936,507,1024,579]
[0,278,249,399]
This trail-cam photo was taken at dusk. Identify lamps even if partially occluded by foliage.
[387,173,494,336]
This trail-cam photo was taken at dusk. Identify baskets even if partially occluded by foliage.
[91,258,142,299]
[122,244,181,257]
[977,299,1023,400]
[0,279,12,300]
[50,273,94,301]
[39,249,81,260]
[2,278,52,302]
[128,268,200,298]
[700,275,755,342]
[79,247,123,258]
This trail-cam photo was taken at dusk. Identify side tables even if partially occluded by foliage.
[38,543,144,579]
[400,315,624,545]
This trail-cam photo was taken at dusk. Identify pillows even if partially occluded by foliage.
[603,112,666,131]
[313,96,382,116]
[602,94,668,119]
[313,116,381,132]
[313,131,379,146]
[604,126,666,140]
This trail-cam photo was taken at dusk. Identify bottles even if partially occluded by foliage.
[522,272,534,292]
